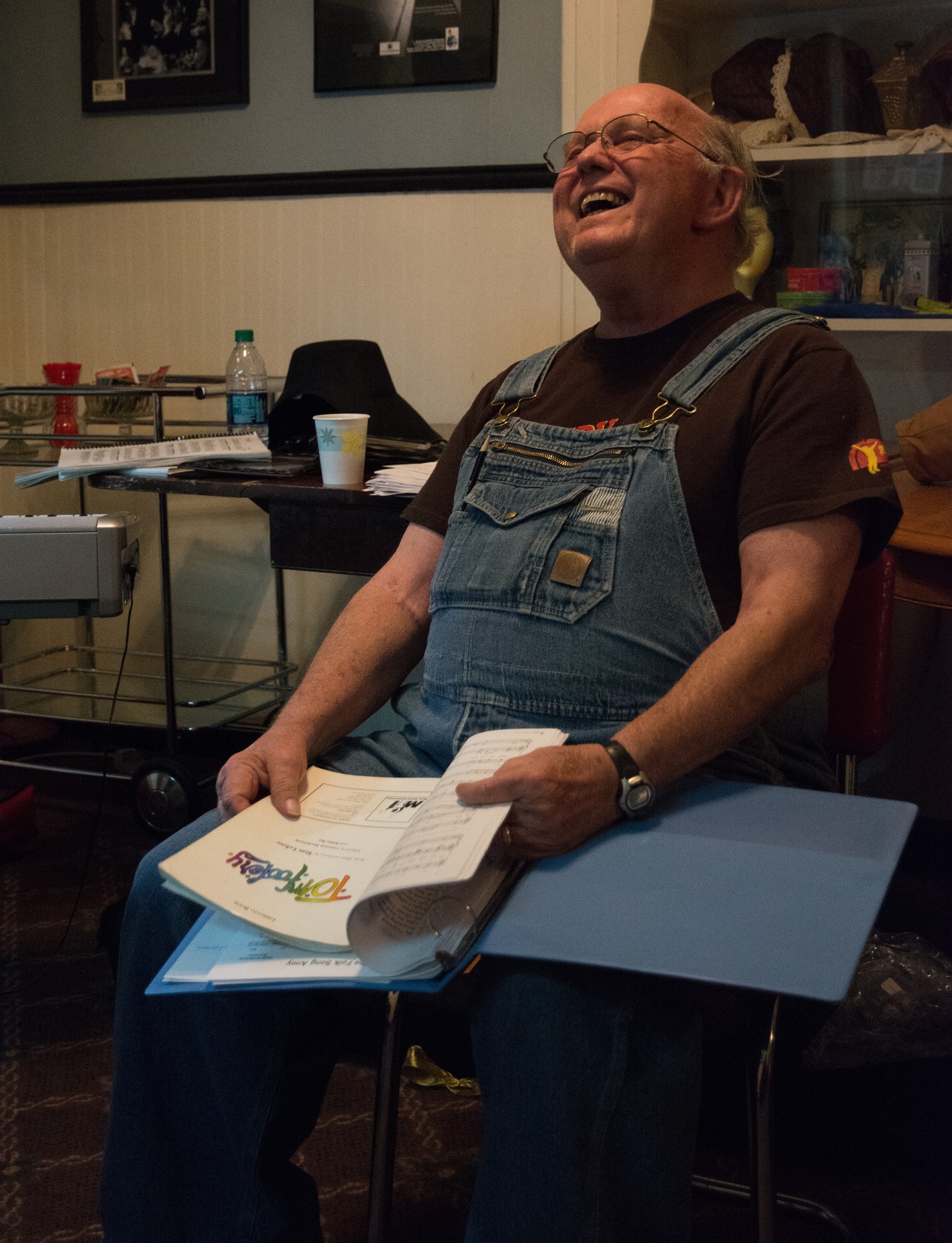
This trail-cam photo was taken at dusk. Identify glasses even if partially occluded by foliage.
[542,112,722,173]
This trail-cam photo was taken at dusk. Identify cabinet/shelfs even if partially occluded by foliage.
[0,374,299,836]
[748,141,952,331]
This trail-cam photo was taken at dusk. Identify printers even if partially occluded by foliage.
[0,509,141,629]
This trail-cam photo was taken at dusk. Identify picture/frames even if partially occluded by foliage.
[79,0,250,115]
[312,0,499,95]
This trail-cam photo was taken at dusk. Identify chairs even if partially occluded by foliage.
[363,543,901,1243]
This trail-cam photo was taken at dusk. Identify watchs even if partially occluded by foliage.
[602,741,657,821]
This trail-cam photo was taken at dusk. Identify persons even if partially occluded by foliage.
[102,81,910,1243]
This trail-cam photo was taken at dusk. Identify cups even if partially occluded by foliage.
[313,413,370,485]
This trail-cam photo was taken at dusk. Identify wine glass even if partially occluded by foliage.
[77,376,164,438]
[0,383,54,460]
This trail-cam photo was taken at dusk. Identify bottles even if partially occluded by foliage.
[225,329,269,450]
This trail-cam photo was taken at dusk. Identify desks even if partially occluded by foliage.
[88,454,441,836]
